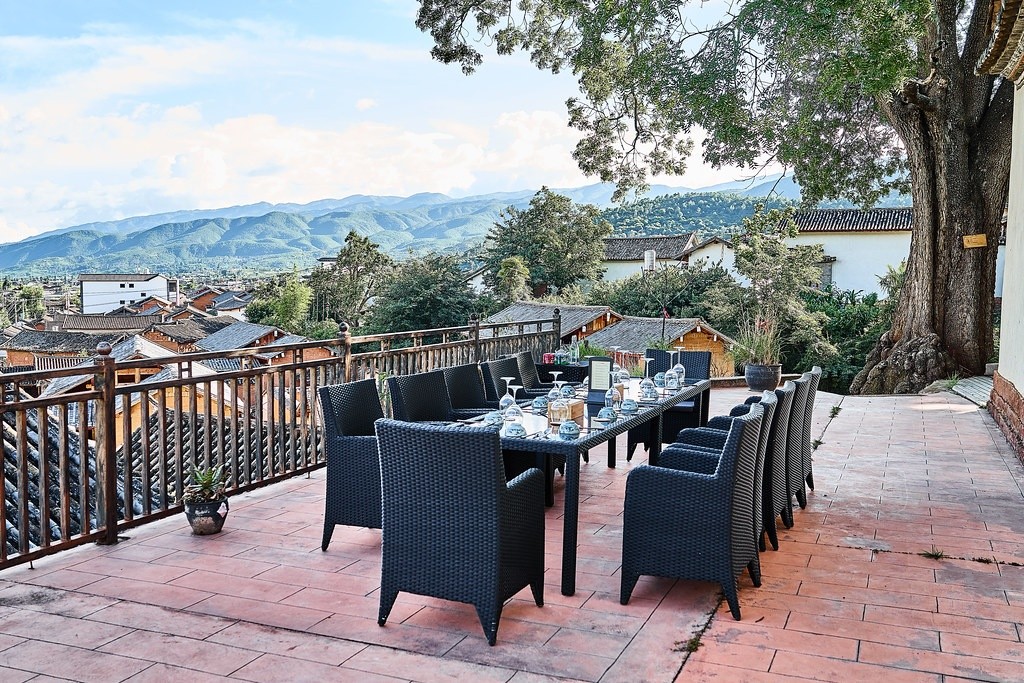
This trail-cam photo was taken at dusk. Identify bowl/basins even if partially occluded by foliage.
[531,396,549,407]
[561,386,576,394]
[505,423,526,436]
[621,399,638,411]
[654,380,667,387]
[654,372,668,380]
[597,407,617,419]
[644,389,659,397]
[558,420,580,433]
[559,433,579,441]
[484,412,503,424]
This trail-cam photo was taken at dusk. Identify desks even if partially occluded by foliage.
[537,360,589,382]
[448,376,711,596]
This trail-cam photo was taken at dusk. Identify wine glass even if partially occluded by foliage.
[499,376,516,416]
[505,385,524,422]
[673,346,685,382]
[610,346,620,382]
[618,350,631,389]
[582,355,596,386]
[551,381,569,423]
[606,372,621,408]
[665,351,678,388]
[640,358,655,390]
[548,371,563,402]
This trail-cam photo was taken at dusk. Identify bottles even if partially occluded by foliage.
[570,335,579,365]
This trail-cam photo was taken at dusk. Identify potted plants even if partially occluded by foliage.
[178,461,233,536]
[734,302,789,391]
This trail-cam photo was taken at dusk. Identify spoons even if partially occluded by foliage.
[579,424,604,430]
[521,428,551,439]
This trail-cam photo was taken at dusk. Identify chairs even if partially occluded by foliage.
[318,351,546,647]
[620,348,823,621]
